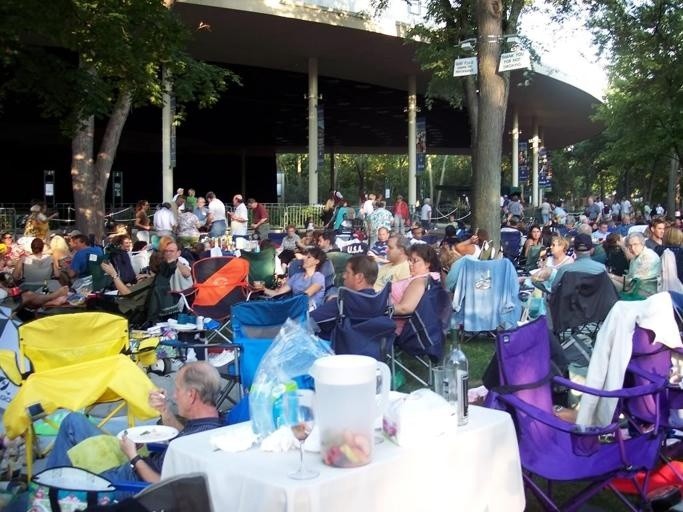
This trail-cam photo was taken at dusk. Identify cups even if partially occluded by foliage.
[178,314,189,324]
[433,366,455,417]
[190,315,195,325]
[195,316,204,330]
[306,355,393,468]
[253,281,264,289]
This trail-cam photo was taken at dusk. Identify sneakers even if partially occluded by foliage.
[209,347,239,368]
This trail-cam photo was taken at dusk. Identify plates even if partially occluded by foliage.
[115,424,179,442]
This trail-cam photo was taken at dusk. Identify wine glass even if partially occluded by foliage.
[281,393,315,480]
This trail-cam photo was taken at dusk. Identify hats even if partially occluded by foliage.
[573,232,598,252]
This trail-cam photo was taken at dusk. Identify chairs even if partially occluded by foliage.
[3,311,169,486]
[178,248,356,345]
[330,282,398,391]
[392,277,454,389]
[30,394,248,511]
[232,293,331,395]
[500,232,522,269]
[441,257,514,344]
[18,280,60,294]
[66,248,138,295]
[482,271,683,512]
[518,245,545,276]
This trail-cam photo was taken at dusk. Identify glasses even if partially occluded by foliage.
[407,258,421,265]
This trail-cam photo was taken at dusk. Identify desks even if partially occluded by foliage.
[168,388,510,512]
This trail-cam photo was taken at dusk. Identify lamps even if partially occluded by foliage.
[455,34,524,54]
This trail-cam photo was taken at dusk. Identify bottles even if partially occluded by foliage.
[6,281,21,304]
[41,279,48,295]
[444,327,468,424]
